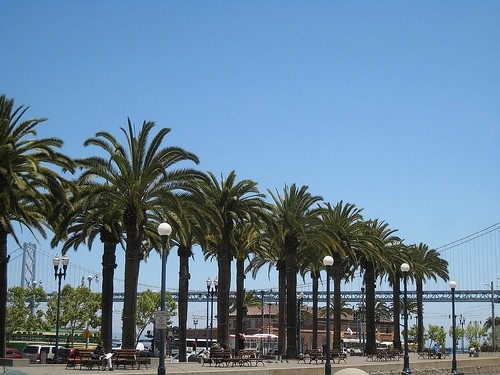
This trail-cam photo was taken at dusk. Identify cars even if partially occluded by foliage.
[5,348,21,359]
[54,347,92,363]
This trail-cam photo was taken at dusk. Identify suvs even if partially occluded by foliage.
[21,344,65,363]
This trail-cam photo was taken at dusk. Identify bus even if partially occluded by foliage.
[235,333,278,355]
[341,338,380,354]
[172,337,216,356]
[377,341,393,352]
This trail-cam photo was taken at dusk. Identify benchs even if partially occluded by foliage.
[297,348,347,364]
[65,348,151,371]
[202,350,265,367]
[366,348,451,362]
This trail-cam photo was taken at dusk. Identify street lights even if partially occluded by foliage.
[53,253,69,362]
[297,292,305,354]
[449,281,458,373]
[156,223,173,375]
[400,262,412,375]
[253,286,273,334]
[485,281,496,352]
[323,255,335,375]
[25,280,43,314]
[193,319,199,354]
[267,302,273,334]
[360,286,365,352]
[80,273,99,349]
[205,276,218,350]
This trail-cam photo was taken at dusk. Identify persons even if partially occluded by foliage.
[211,343,232,364]
[433,342,445,359]
[94,341,113,370]
[469,345,478,357]
[59,347,67,363]
